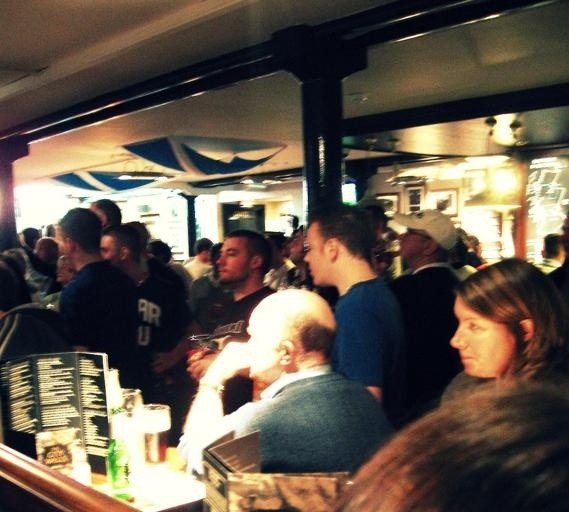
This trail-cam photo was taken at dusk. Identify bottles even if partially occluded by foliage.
[105,408,135,505]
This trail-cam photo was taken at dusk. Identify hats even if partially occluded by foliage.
[395,209,457,250]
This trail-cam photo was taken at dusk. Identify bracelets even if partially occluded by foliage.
[199,378,225,392]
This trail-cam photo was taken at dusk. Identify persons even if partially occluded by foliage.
[1,199,340,446]
[179,288,398,478]
[450,257,567,383]
[329,378,566,510]
[302,206,411,422]
[357,203,569,429]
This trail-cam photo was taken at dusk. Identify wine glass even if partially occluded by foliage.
[188,333,215,399]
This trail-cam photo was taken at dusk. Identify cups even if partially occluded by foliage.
[121,388,145,420]
[374,242,403,278]
[137,404,172,465]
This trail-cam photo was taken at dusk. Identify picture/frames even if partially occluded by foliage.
[374,183,459,219]
